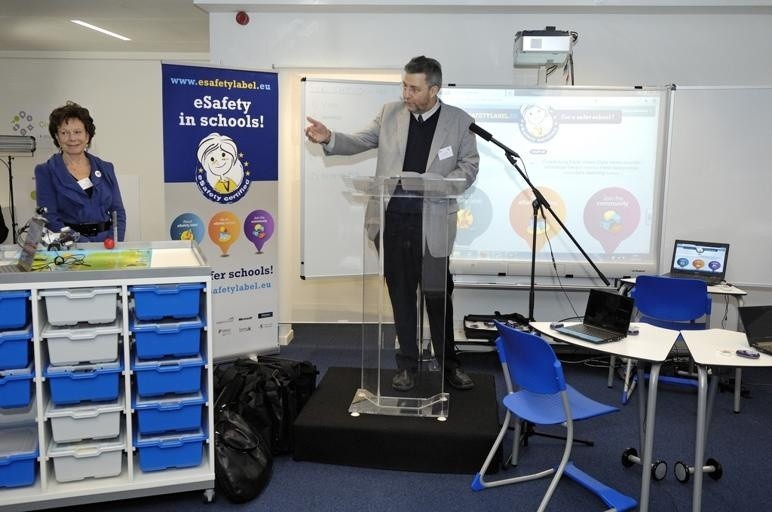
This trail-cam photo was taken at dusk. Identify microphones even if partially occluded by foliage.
[469,123,519,157]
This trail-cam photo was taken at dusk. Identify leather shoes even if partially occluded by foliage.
[441,365,474,390]
[392,368,416,391]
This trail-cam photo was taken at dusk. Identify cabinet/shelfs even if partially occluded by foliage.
[0,240,217,512]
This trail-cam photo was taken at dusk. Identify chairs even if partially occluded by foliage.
[470,316,639,512]
[622,275,713,407]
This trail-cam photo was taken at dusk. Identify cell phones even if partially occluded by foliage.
[737,350,760,359]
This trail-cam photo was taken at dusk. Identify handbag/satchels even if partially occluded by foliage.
[214,404,274,504]
[213,355,319,456]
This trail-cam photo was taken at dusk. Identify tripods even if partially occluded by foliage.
[499,155,610,471]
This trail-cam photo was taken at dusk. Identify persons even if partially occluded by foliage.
[34,100,126,243]
[304,55,480,391]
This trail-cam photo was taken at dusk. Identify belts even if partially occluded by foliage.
[64,221,111,237]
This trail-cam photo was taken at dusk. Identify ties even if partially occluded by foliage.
[418,115,423,123]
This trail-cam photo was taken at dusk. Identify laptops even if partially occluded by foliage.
[556,289,634,344]
[738,306,772,356]
[662,240,729,285]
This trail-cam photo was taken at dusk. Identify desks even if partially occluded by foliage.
[679,328,772,512]
[606,276,748,413]
[528,319,681,512]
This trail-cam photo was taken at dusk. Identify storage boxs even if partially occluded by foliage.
[3,429,40,487]
[46,402,122,444]
[3,333,31,371]
[136,433,205,473]
[1,375,33,409]
[132,285,202,321]
[138,401,205,435]
[44,325,122,366]
[49,441,124,483]
[40,288,122,326]
[3,292,30,329]
[44,364,122,404]
[131,324,205,360]
[136,363,204,398]
